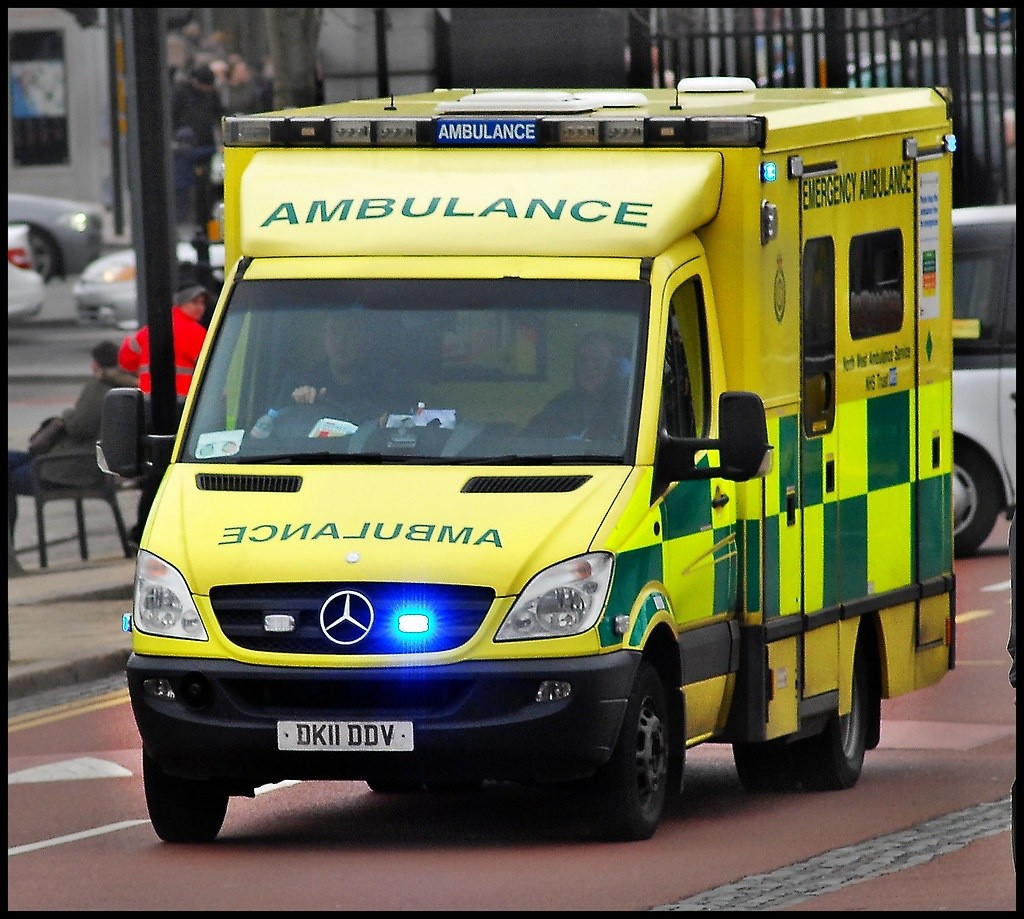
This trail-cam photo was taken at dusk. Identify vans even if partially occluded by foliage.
[949,203,1017,558]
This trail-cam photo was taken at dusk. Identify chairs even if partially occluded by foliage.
[27,387,144,570]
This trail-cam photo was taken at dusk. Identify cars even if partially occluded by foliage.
[72,235,233,330]
[6,224,44,322]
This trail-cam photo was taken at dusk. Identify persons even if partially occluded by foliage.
[275,296,415,460]
[8,342,140,575]
[160,12,277,228]
[119,276,210,552]
[517,331,627,455]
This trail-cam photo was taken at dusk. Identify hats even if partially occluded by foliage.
[174,281,209,305]
[93,341,119,366]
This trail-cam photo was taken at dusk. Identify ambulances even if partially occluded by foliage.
[95,73,955,843]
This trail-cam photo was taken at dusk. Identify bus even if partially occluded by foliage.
[7,8,132,282]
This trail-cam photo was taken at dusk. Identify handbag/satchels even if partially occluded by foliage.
[29,416,64,456]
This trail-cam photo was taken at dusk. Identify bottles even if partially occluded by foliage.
[251,409,279,440]
